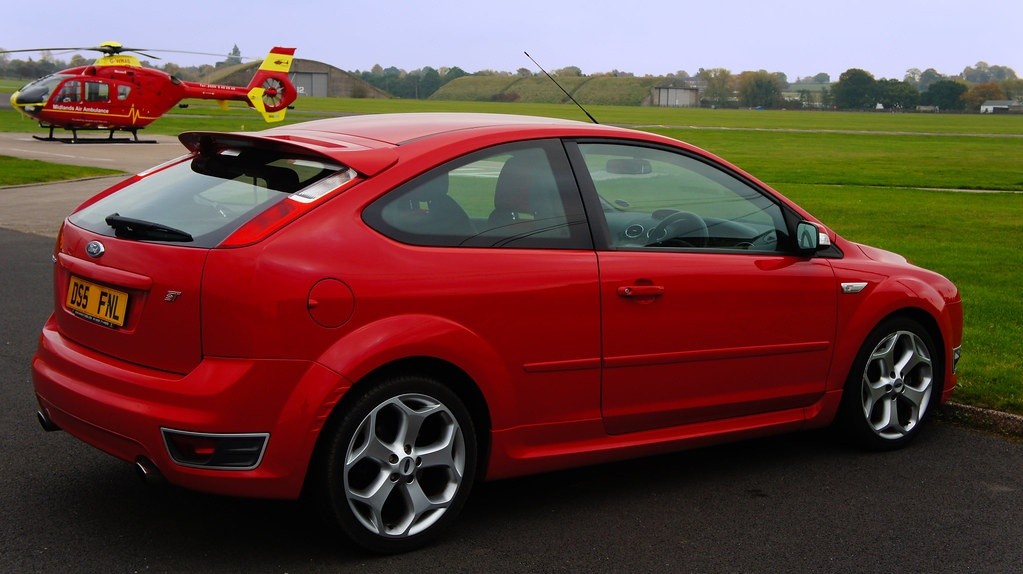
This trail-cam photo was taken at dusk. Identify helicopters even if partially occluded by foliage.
[0,40,298,143]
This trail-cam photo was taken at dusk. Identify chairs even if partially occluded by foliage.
[409,171,472,226]
[484,158,562,239]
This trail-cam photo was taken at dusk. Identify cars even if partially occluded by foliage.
[34,112,963,559]
